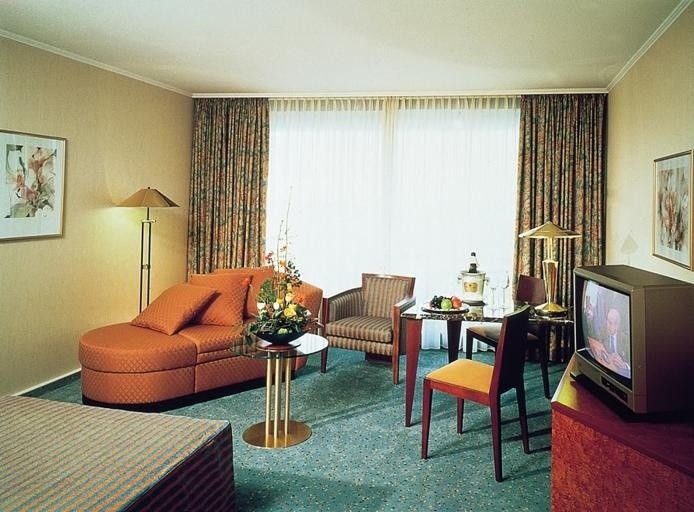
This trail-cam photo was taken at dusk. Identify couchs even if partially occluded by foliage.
[78,280,323,413]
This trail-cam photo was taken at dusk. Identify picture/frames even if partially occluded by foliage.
[0,129,68,243]
[652,149,694,271]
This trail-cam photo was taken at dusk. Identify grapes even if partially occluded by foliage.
[430,295,451,309]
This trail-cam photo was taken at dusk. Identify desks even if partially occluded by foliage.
[400,303,574,427]
[230,332,329,449]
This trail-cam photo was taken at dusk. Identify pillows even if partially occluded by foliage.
[213,265,274,319]
[190,274,252,326]
[131,282,216,336]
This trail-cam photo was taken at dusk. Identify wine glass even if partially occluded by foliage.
[486,271,510,309]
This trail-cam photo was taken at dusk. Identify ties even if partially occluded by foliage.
[610,336,614,354]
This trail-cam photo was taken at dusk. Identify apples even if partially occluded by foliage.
[452,299,462,309]
[440,299,452,310]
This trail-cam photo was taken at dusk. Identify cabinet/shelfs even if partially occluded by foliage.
[550,353,693,512]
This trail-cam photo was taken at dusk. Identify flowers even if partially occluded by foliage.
[230,186,324,347]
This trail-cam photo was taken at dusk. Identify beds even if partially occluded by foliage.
[0,394,235,512]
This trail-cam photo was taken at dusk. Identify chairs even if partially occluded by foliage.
[321,273,416,385]
[466,275,549,398]
[421,304,532,482]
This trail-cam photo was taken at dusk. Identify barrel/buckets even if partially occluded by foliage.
[461,271,486,303]
[462,300,483,322]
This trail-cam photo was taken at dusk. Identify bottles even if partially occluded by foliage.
[468,252,479,273]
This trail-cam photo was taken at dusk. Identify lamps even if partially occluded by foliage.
[518,221,581,313]
[117,187,180,313]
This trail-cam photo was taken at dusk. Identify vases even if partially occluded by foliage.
[252,328,310,345]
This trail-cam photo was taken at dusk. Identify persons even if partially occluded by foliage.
[579,295,631,378]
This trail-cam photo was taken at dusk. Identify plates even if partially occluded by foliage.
[423,301,470,313]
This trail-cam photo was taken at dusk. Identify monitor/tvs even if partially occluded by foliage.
[569,264,694,424]
